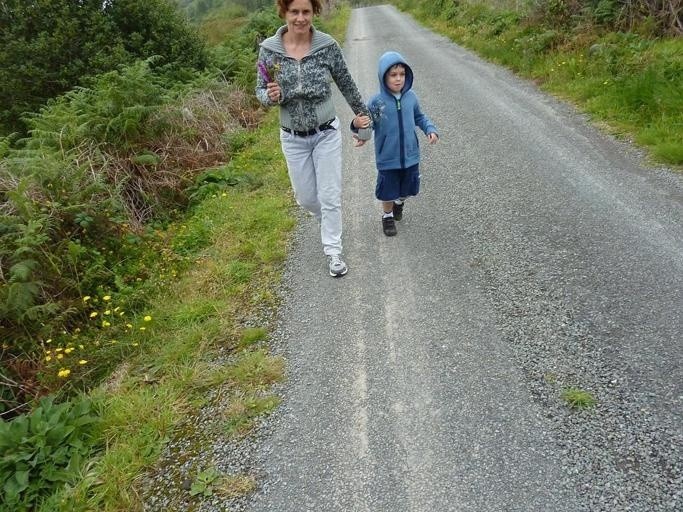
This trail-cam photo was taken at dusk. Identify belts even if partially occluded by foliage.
[281,117,336,136]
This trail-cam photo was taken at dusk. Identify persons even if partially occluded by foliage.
[350,50,439,236]
[254,0,373,278]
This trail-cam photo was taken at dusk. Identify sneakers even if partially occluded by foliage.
[326,254,348,277]
[382,201,404,236]
